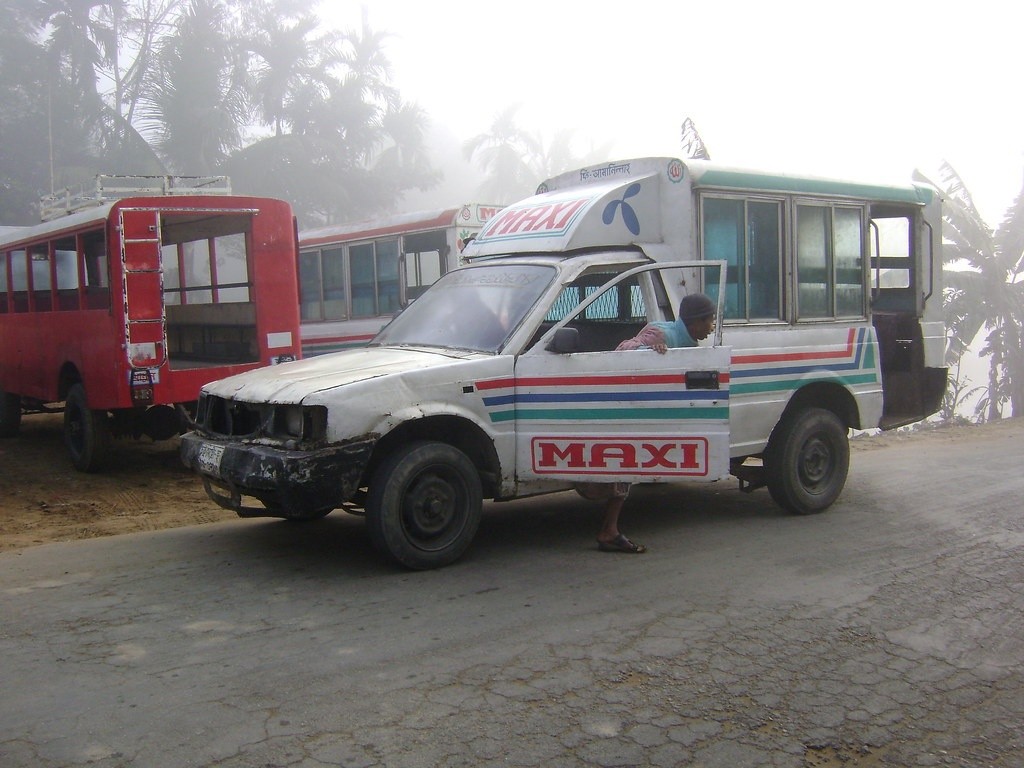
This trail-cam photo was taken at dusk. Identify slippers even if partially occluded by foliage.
[598,529,648,554]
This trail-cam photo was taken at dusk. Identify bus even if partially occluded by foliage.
[0,173,301,476]
[296,202,516,358]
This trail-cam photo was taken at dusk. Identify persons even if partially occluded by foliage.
[596,293,716,553]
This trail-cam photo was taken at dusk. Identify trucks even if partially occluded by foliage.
[174,156,951,573]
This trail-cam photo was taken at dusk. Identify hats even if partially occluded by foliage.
[678,294,717,322]
